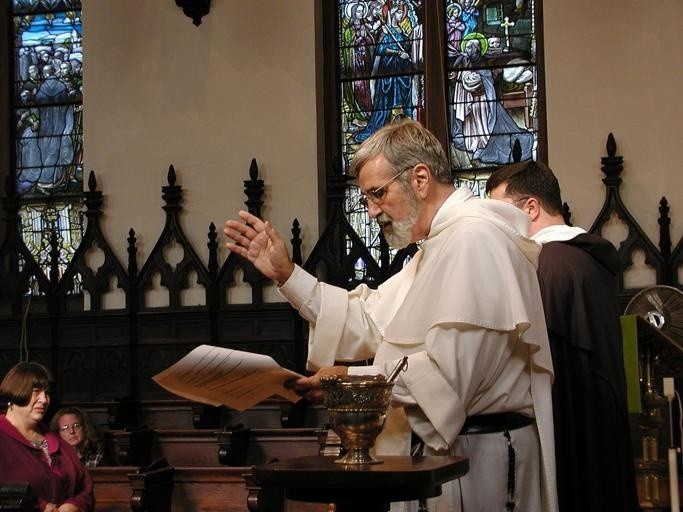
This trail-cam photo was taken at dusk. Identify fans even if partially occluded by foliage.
[623,285,683,349]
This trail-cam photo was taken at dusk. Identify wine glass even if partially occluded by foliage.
[319,373,394,466]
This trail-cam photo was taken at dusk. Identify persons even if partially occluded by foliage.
[49,406,123,467]
[340,0,533,163]
[1,360,97,511]
[480,156,637,512]
[223,119,559,510]
[18,45,84,194]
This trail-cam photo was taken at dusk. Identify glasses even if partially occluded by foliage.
[62,424,82,432]
[362,166,413,203]
